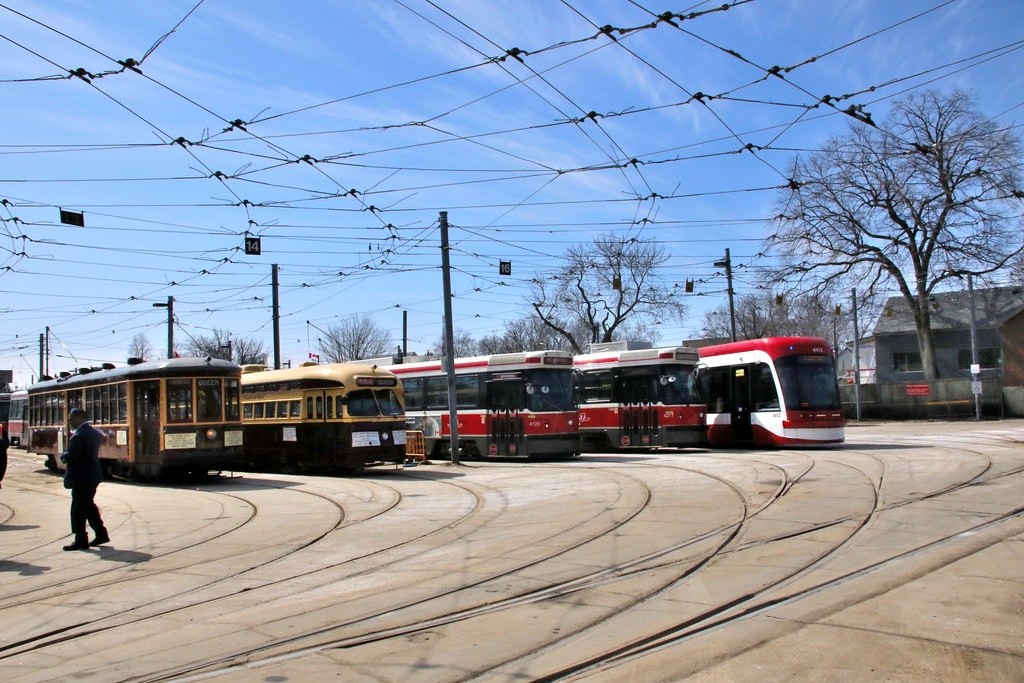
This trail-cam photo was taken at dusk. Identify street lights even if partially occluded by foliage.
[153,296,174,359]
[713,247,737,343]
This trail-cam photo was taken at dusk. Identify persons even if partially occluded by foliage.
[60,408,110,551]
[422,416,440,436]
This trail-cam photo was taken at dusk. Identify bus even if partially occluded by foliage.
[346,342,564,463]
[0,351,243,485]
[696,336,845,450]
[239,361,409,477]
[573,339,710,454]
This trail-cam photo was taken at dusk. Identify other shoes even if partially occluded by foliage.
[62,540,90,550]
[88,536,110,547]
